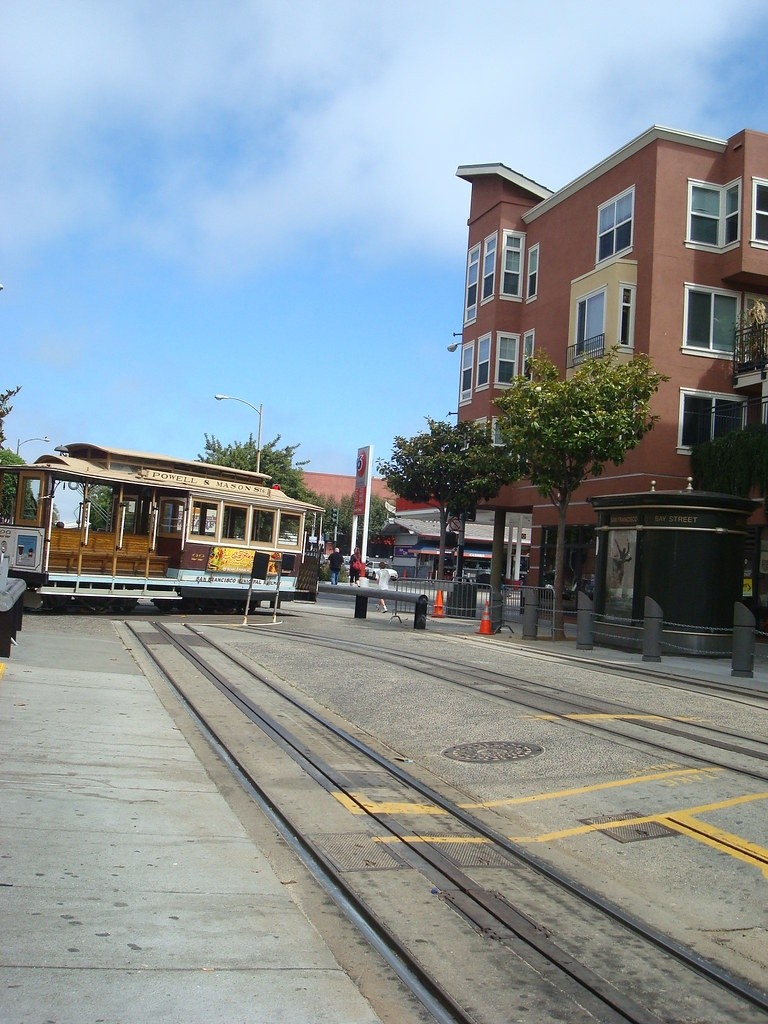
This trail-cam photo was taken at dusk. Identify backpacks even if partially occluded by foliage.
[352,554,363,571]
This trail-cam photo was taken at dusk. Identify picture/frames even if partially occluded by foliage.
[392,545,417,558]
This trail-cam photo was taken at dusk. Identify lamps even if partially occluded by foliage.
[447,343,462,352]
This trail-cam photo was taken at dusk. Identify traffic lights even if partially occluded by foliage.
[272,483,281,491]
[331,507,340,525]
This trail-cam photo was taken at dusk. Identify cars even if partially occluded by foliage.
[570,573,596,600]
[318,553,351,576]
[365,560,399,581]
[475,571,507,591]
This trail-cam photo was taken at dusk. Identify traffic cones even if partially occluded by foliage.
[475,600,495,635]
[431,606,446,618]
[402,567,408,578]
[433,589,445,607]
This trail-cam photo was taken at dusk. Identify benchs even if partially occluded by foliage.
[49,528,170,578]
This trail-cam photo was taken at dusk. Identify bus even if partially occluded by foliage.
[0,442,327,617]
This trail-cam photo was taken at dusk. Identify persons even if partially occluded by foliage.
[375,562,390,613]
[349,547,361,587]
[328,548,344,585]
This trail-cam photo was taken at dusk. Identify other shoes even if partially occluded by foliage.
[381,608,388,613]
[375,604,380,610]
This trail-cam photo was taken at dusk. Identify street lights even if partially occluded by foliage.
[16,436,51,455]
[214,394,263,474]
